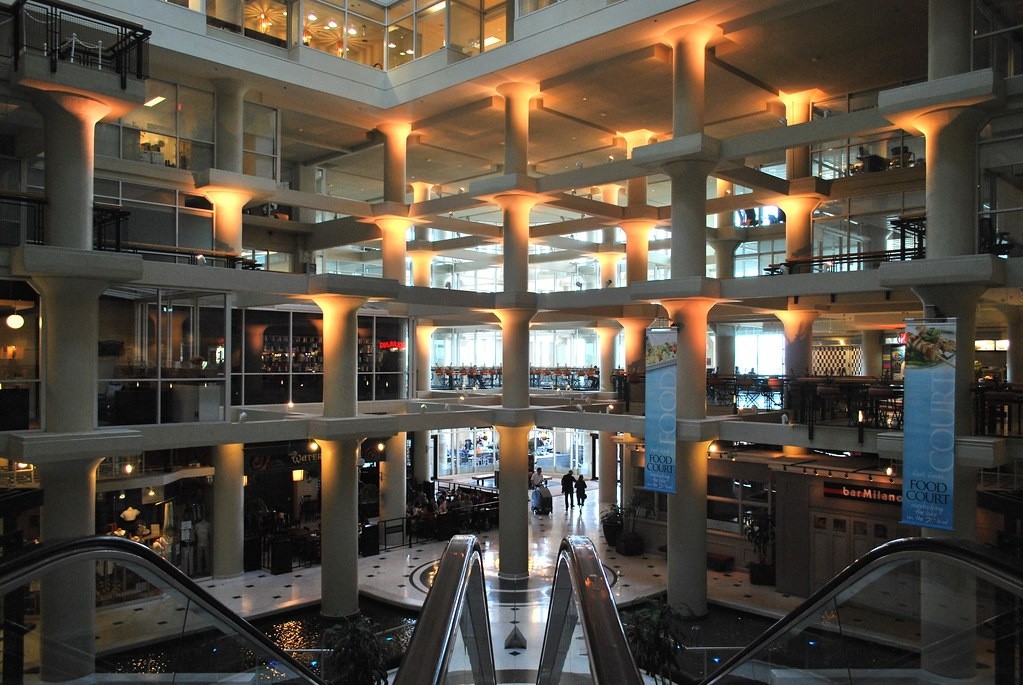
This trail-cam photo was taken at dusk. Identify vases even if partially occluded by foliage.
[604,520,623,546]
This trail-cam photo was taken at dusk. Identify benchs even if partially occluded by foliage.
[472,474,495,487]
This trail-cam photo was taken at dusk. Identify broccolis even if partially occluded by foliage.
[921,327,941,341]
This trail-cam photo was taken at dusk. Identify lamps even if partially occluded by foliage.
[6,298,24,330]
[782,465,895,484]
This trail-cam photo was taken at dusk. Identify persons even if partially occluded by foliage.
[528,467,587,511]
[181,509,210,576]
[468,365,486,387]
[588,366,599,388]
[406,487,488,533]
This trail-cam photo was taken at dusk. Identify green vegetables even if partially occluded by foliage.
[905,347,928,366]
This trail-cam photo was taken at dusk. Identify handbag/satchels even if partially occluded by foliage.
[584,494,587,498]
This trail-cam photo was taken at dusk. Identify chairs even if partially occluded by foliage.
[406,491,492,543]
[98,384,126,425]
[293,530,321,568]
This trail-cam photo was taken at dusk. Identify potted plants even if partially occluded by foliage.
[742,508,776,586]
[614,492,657,557]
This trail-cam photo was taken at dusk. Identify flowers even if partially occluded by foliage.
[598,504,628,525]
